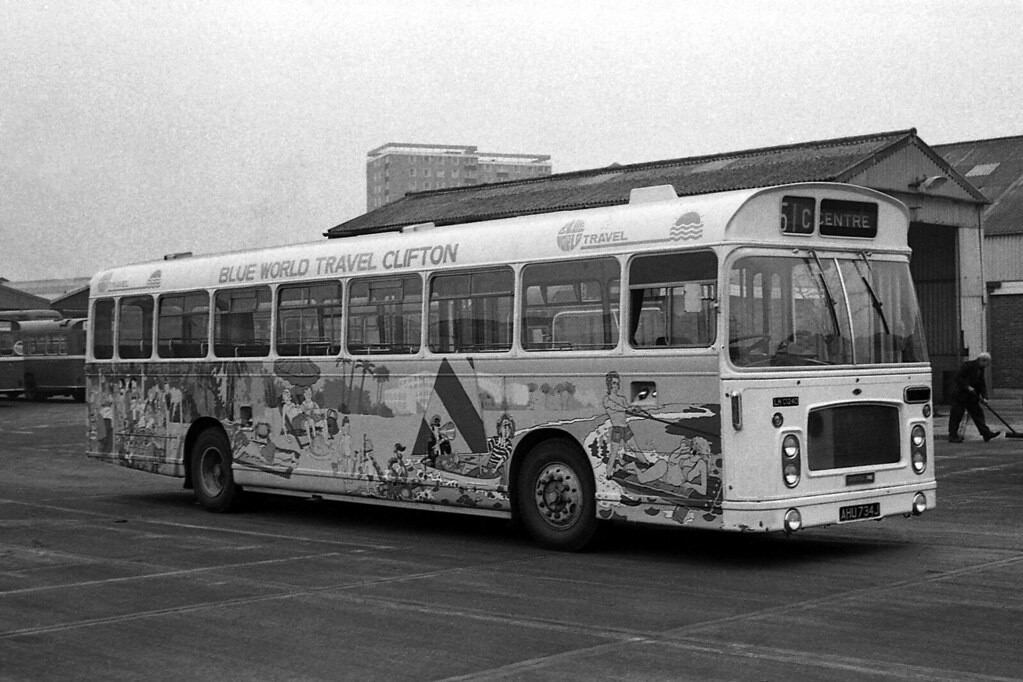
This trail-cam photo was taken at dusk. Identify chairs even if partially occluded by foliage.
[121,311,537,357]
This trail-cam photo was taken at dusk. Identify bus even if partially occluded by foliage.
[84,179,939,556]
[0,308,87,401]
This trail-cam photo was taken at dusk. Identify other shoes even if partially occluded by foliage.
[948,437,963,444]
[981,430,1005,442]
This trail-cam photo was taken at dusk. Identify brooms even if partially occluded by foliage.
[970,390,1023,438]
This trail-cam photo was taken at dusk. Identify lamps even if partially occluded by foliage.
[909,175,948,194]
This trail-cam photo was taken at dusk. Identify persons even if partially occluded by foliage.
[947,352,1002,441]
